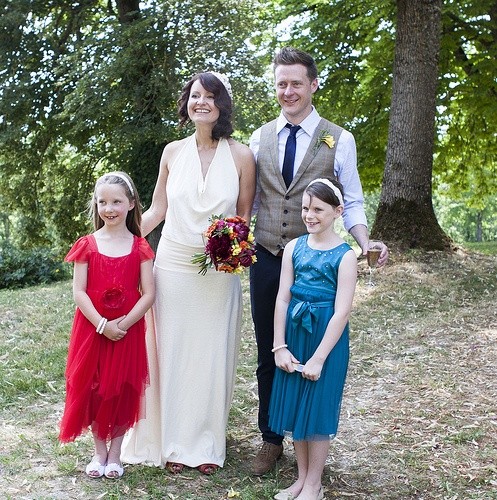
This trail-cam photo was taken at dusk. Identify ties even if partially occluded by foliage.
[281,123,302,190]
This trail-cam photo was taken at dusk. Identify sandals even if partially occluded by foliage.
[166,462,183,472]
[86,454,105,479]
[105,456,123,480]
[197,463,219,476]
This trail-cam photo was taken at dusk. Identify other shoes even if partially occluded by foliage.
[273,480,307,500]
[292,486,324,500]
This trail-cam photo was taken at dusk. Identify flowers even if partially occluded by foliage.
[96,285,128,311]
[316,129,335,149]
[190,213,258,276]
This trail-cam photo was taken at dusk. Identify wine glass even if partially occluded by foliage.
[365,240,383,287]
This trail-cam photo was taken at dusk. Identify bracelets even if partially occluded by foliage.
[271,343,288,353]
[96,317,108,335]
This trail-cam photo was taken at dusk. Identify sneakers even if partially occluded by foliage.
[250,442,283,475]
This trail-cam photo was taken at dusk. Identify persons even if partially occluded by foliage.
[244,46,388,477]
[56,169,157,478]
[117,72,258,476]
[273,177,358,500]
